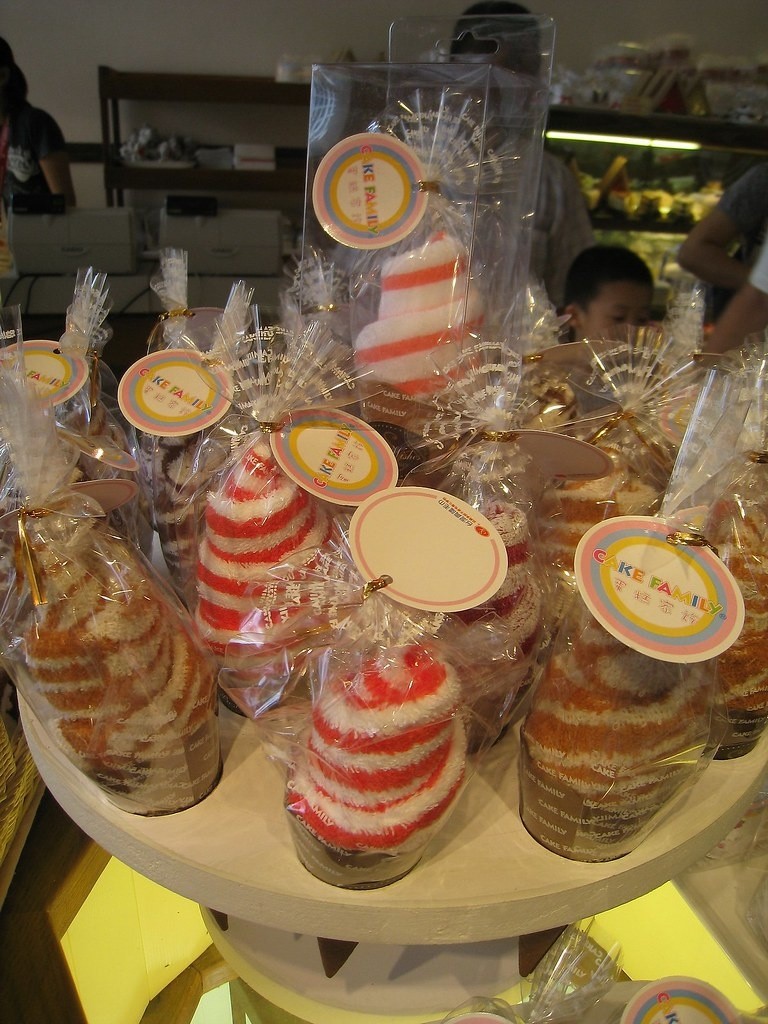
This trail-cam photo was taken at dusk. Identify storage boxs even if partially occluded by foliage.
[232,143,275,170]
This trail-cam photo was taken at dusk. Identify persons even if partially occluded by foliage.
[0,36,77,208]
[347,0,768,365]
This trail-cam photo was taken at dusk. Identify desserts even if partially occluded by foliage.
[0,233,768,889]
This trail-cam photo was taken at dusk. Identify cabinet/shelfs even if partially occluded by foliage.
[84,66,768,234]
